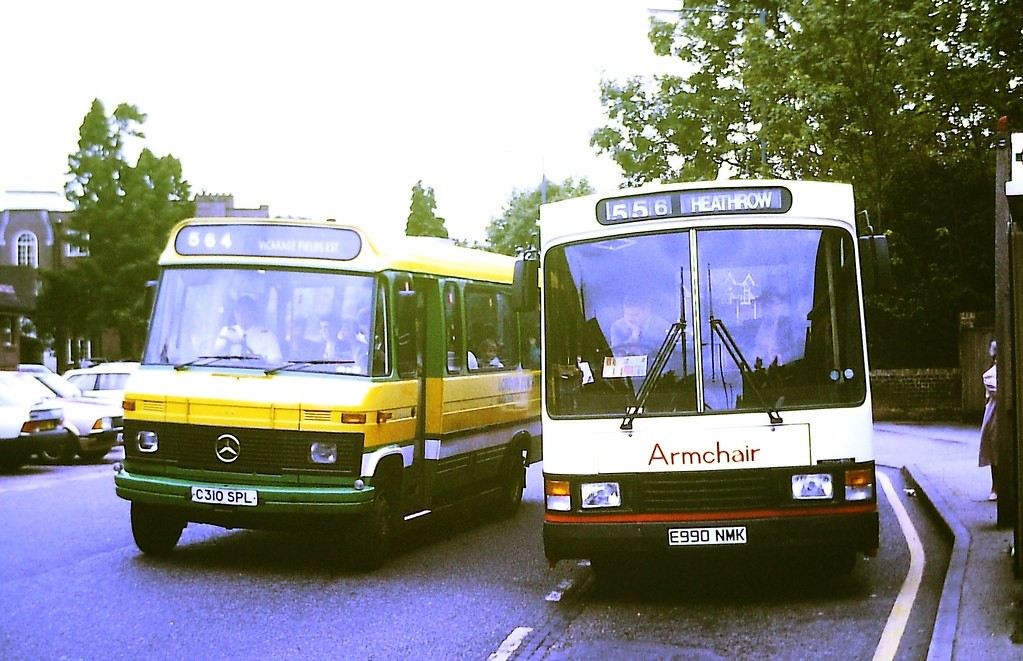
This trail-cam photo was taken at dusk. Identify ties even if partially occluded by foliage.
[241,334,247,357]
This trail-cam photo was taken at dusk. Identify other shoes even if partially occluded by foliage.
[989,491,998,502]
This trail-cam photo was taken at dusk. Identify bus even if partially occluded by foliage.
[111,213,544,568]
[507,178,880,602]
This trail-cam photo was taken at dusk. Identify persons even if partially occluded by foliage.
[215,295,283,362]
[741,286,794,353]
[447,330,478,370]
[978,341,1000,501]
[476,340,505,369]
[610,290,675,348]
[288,307,372,368]
[523,336,541,368]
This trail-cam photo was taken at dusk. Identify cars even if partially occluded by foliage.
[0,358,139,473]
